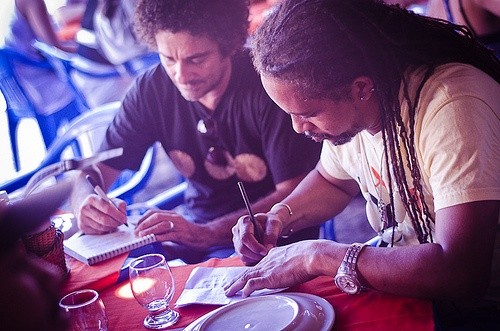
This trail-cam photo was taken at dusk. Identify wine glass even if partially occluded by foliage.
[128,253,180,330]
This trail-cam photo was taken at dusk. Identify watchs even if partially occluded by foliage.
[334,242,371,295]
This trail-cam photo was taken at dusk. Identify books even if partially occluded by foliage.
[63,222,155,267]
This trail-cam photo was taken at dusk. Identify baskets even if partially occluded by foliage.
[38,229,69,278]
[25,221,57,257]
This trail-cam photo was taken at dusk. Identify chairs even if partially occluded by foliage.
[0,47,188,215]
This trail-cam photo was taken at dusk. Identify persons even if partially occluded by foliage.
[0,0,500,331]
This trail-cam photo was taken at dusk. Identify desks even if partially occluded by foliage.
[51,209,435,331]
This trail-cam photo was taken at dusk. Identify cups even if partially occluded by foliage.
[58,289,109,331]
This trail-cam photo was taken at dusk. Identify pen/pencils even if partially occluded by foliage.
[85,175,129,229]
[238,181,263,245]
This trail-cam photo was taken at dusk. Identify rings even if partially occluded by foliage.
[168,221,174,232]
[236,236,240,245]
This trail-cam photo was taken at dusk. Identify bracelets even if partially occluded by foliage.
[270,202,293,238]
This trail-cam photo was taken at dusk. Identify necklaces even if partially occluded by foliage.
[359,132,387,223]
[350,129,410,243]
[197,109,214,133]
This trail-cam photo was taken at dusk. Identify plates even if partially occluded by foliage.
[182,292,335,331]
